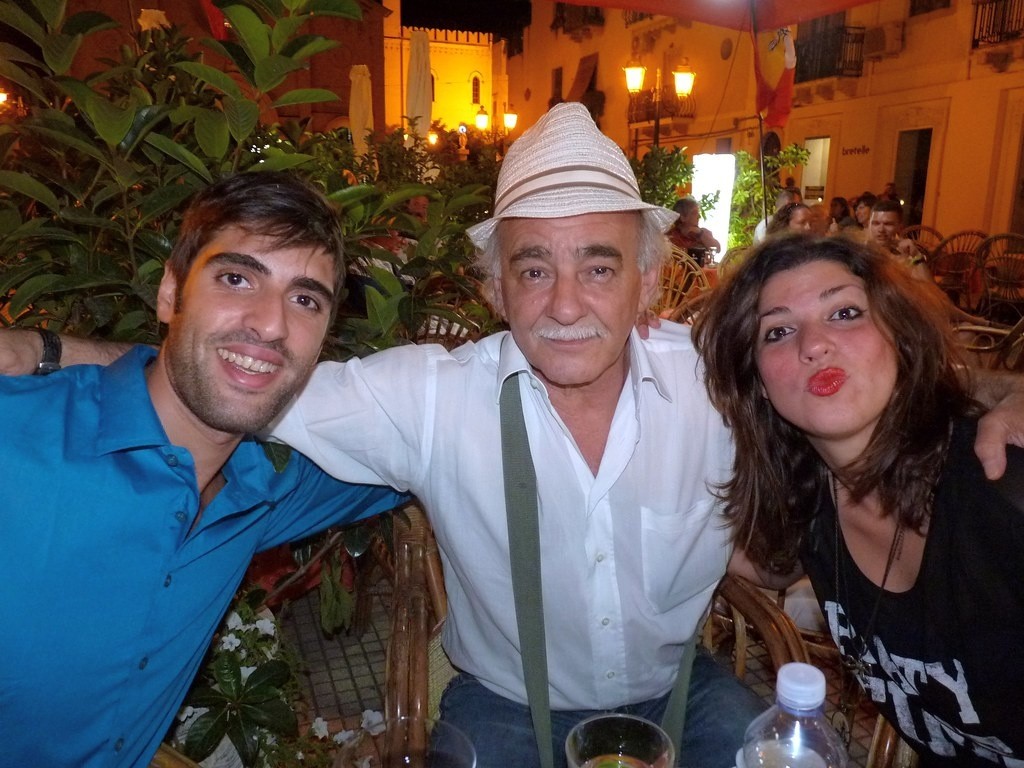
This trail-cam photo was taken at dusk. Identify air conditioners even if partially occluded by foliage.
[862,19,905,58]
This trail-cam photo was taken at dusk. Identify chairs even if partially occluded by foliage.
[975,232,1024,324]
[928,229,993,312]
[383,500,812,768]
[954,317,1024,373]
[899,224,948,276]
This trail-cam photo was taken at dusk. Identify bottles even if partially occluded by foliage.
[743,663,850,768]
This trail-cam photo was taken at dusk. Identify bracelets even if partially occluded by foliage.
[5,322,64,376]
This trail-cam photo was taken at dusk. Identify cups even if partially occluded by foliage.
[565,713,674,768]
[333,715,477,768]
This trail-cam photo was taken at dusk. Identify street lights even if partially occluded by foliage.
[473,98,519,214]
[622,57,698,169]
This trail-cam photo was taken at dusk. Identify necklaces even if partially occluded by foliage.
[828,478,902,746]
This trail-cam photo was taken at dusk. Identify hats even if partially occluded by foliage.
[464,102,681,252]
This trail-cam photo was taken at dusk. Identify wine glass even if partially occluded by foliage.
[709,246,718,269]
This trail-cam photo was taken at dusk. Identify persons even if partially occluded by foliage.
[0,103,1024,768]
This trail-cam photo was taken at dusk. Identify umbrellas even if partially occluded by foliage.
[555,0,879,126]
